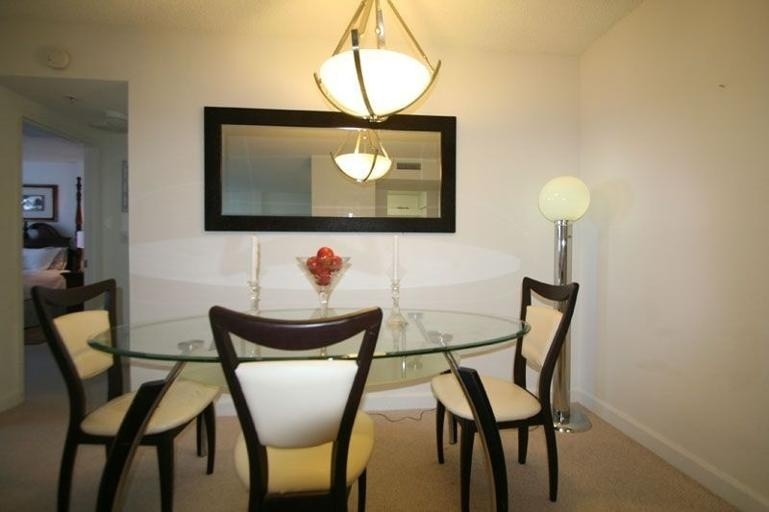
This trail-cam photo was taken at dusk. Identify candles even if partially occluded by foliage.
[393,236,399,281]
[251,237,260,281]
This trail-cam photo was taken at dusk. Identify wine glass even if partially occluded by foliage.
[297,256,353,318]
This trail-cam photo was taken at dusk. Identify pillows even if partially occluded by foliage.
[20,246,61,273]
[47,246,68,270]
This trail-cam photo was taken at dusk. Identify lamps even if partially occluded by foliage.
[538,175,593,433]
[330,129,394,185]
[313,0,442,125]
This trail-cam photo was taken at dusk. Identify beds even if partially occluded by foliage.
[22,221,85,344]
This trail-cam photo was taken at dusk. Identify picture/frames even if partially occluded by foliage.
[22,184,57,220]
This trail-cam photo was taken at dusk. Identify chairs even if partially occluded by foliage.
[430,278,579,511]
[209,304,383,512]
[30,279,221,512]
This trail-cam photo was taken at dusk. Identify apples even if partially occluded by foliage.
[308,246,343,285]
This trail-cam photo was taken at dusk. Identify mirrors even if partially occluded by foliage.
[203,106,457,233]
